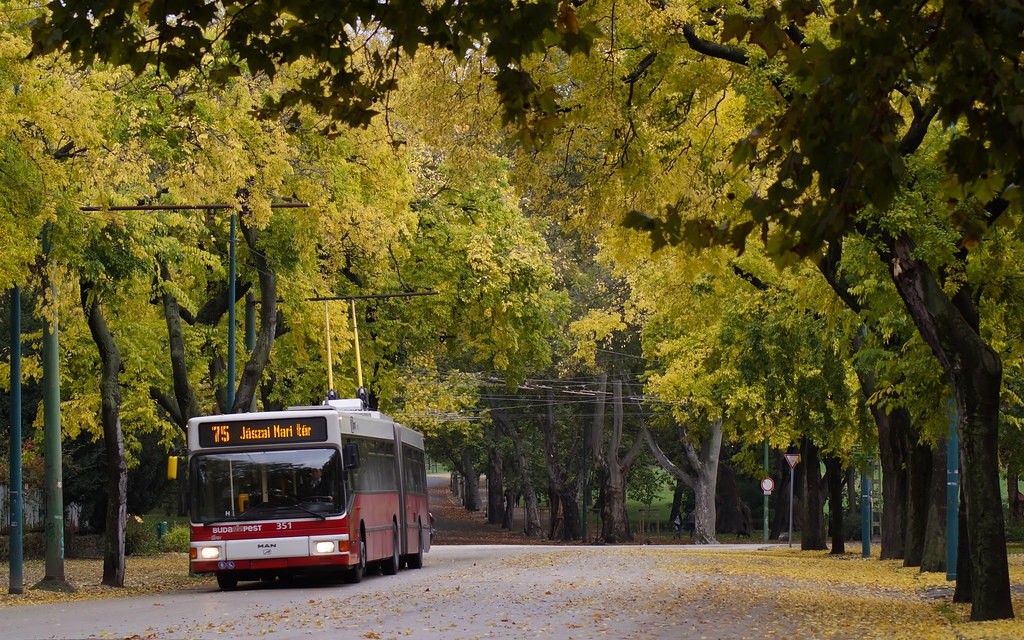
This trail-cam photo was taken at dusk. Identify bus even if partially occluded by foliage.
[184,398,434,590]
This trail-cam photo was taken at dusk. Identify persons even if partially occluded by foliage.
[688,508,696,538]
[673,510,682,539]
[300,469,339,502]
[429,511,437,545]
